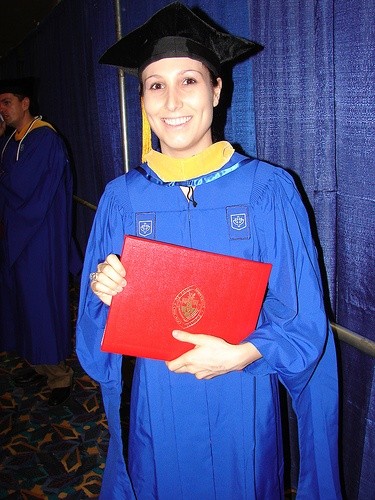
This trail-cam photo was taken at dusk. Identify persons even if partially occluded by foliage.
[0,73,75,406]
[76,0,341,500]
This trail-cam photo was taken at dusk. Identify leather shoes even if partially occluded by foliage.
[47,387,72,408]
[13,369,48,386]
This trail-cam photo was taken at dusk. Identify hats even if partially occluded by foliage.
[0,76,36,95]
[97,0,262,164]
